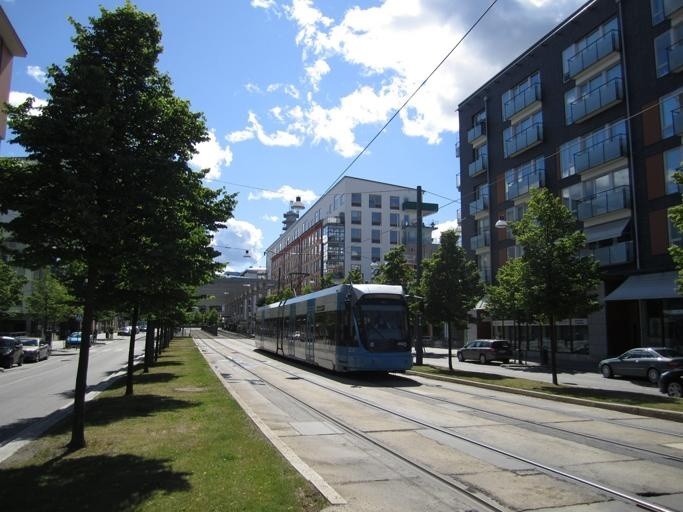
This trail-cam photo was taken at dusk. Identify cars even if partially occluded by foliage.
[18,336,49,362]
[0,335,24,368]
[412,336,431,345]
[457,338,512,364]
[659,367,682,398]
[117,326,139,336]
[66,331,93,347]
[598,346,683,383]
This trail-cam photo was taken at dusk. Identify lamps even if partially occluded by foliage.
[290,196,307,210]
[242,249,252,257]
[494,215,507,229]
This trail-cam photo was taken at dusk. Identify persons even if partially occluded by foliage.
[374,316,392,330]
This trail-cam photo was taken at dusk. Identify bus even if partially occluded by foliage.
[255,283,412,373]
